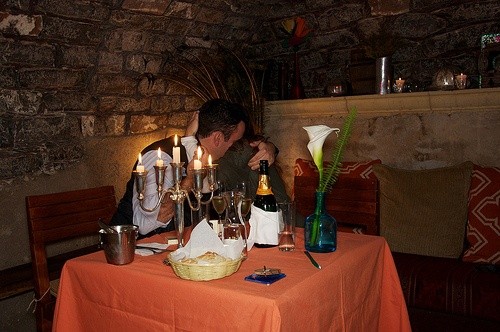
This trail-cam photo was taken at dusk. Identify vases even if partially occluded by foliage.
[291,53,305,99]
[375,56,391,93]
[304,189,338,254]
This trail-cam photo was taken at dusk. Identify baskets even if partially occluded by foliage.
[167,250,244,282]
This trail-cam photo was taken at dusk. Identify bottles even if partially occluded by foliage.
[254,160,279,248]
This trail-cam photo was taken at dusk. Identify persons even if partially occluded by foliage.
[108,98,280,241]
[180,109,306,227]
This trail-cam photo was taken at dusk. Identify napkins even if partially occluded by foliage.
[135,240,168,257]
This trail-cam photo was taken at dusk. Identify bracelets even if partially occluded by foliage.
[268,141,280,160]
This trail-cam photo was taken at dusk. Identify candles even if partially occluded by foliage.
[137,152,144,172]
[156,147,164,167]
[172,134,181,164]
[194,146,203,169]
[208,155,214,166]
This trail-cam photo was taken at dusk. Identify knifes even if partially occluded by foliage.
[304,251,321,269]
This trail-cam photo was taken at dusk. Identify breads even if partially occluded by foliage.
[176,251,221,265]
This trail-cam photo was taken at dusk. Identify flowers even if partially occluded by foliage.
[302,110,359,190]
[274,17,321,51]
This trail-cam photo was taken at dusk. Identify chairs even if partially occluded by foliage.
[27,185,117,332]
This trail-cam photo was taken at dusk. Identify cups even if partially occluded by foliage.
[277,201,296,243]
[98,225,139,265]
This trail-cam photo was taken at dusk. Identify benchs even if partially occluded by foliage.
[292,157,500,332]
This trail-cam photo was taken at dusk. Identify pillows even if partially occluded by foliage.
[462,162,500,265]
[376,161,476,258]
[295,159,382,180]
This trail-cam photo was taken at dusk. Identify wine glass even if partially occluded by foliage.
[211,181,250,228]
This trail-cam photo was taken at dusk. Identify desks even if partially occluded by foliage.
[51,222,414,332]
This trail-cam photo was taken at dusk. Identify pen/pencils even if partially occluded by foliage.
[304,250,322,270]
